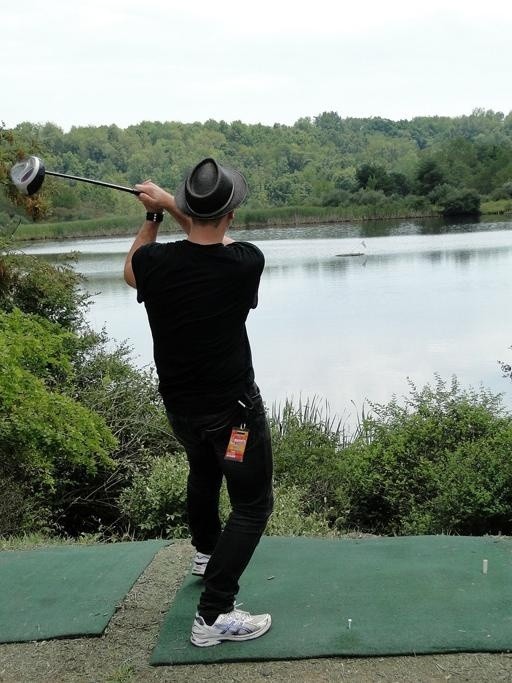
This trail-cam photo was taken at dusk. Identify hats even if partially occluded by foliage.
[174,158,249,220]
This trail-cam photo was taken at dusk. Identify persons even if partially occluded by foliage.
[124,158,275,648]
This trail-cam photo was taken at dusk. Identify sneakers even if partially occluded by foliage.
[191,552,212,575]
[190,601,272,648]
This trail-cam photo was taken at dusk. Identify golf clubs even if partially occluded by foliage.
[11,154,139,196]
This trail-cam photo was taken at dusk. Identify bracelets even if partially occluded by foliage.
[146,212,165,224]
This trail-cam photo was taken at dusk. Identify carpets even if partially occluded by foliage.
[148,535,511,666]
[0,539,174,644]
